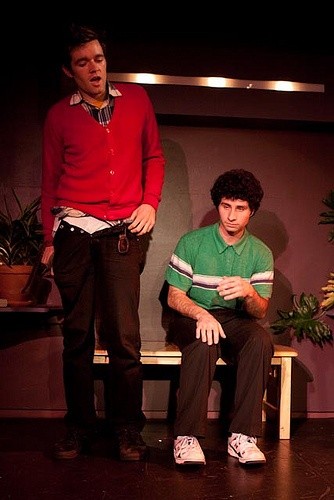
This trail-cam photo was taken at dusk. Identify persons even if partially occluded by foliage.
[41,25,167,462]
[163,167,276,466]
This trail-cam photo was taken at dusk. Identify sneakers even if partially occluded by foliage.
[173,436,206,464]
[227,432,266,463]
[55,430,88,460]
[117,426,147,460]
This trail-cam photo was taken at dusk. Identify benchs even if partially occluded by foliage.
[91,341,299,440]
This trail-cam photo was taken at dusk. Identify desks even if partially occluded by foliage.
[0,303,63,315]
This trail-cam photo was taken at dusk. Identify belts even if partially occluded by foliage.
[117,224,129,253]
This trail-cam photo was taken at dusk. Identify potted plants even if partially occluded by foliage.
[0,184,49,307]
